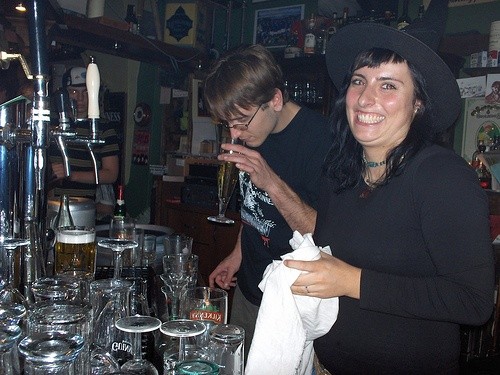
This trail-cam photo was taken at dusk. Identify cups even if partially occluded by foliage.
[0,217,246,375]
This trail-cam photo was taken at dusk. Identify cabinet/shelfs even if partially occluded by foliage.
[170,204,240,299]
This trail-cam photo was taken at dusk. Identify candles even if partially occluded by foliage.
[87,0,105,18]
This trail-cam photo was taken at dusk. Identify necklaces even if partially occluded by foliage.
[361,150,386,167]
[359,168,386,198]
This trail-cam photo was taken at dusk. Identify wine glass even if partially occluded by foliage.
[160,272,186,292]
[161,284,186,320]
[0,238,31,310]
[97,238,139,280]
[206,137,246,224]
[160,319,207,375]
[115,315,162,375]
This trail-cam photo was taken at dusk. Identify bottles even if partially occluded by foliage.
[109,185,135,268]
[283,0,424,109]
[53,191,75,231]
[471,134,500,189]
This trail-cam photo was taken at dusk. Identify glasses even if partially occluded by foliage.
[216,103,263,130]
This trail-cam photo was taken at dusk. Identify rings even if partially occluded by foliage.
[305,286,309,293]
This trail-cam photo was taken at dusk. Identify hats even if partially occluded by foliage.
[325,0,462,141]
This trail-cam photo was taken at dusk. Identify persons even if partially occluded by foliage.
[48,65,120,199]
[283,26,495,375]
[202,46,329,367]
